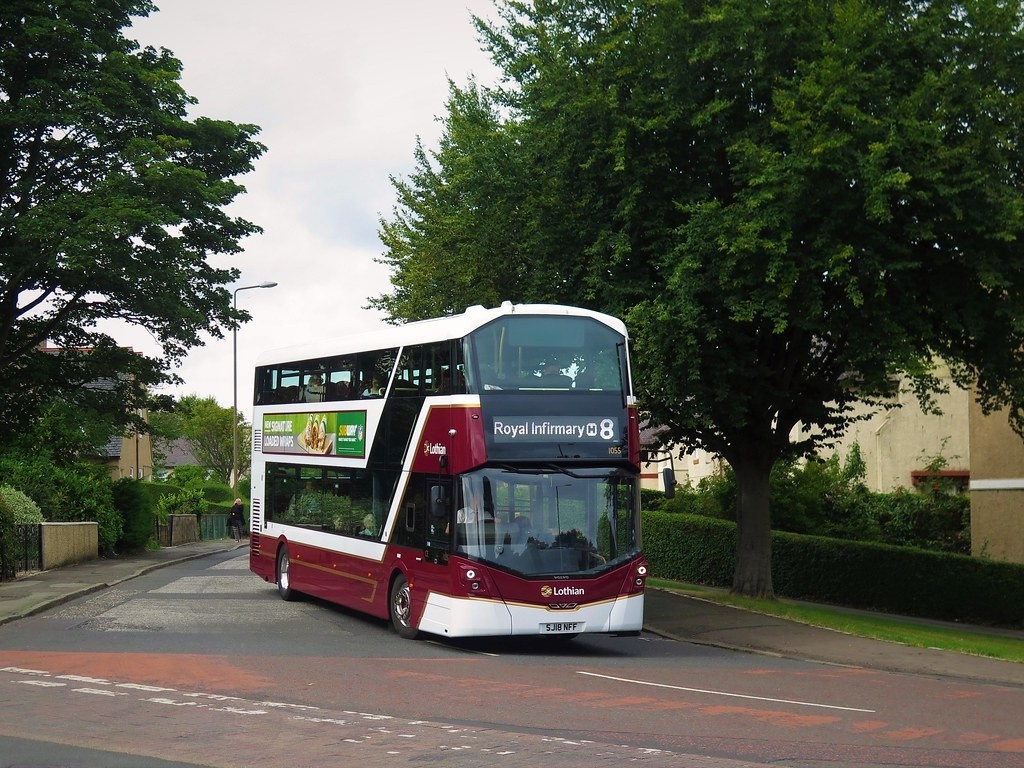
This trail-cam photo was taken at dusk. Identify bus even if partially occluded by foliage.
[247,299,678,641]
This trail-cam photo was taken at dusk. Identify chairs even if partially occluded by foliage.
[272,379,460,398]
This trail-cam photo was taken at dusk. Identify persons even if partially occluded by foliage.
[226,497,246,543]
[443,489,502,560]
[299,368,388,400]
[287,477,380,538]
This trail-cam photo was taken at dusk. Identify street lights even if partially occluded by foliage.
[233,281,278,507]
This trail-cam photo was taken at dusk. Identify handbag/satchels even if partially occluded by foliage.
[227,519,232,527]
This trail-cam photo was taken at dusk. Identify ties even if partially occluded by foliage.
[473,512,478,524]
